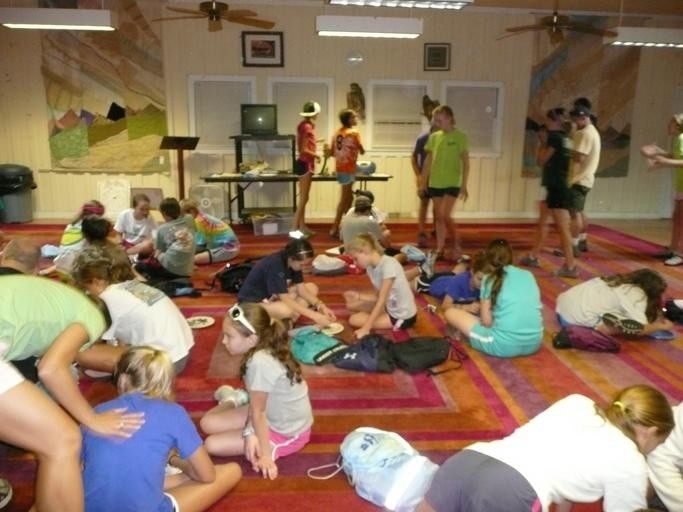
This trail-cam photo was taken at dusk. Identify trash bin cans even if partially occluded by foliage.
[0,163,36,224]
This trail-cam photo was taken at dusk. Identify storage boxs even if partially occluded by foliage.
[252,214,297,236]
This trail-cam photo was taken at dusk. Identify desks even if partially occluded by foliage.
[202,166,395,230]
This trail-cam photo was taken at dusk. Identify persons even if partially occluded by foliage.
[199,301,314,481]
[641,114,683,266]
[0,274,146,512]
[1,191,683,403]
[328,109,365,238]
[288,99,321,240]
[79,347,243,512]
[411,384,674,512]
[411,105,470,261]
[519,97,602,277]
[645,401,683,512]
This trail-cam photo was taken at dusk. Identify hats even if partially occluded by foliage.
[354,191,373,208]
[298,100,320,118]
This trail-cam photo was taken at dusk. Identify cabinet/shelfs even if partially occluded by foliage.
[229,131,296,219]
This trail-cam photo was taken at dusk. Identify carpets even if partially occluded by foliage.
[1,224,682,512]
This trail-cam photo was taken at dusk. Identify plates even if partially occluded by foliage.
[650,330,676,339]
[186,316,215,330]
[314,322,344,336]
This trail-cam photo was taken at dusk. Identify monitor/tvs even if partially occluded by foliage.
[239,103,278,139]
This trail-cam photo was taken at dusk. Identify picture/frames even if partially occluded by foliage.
[423,41,451,73]
[241,30,284,69]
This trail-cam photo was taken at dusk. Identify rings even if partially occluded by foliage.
[120,422,124,428]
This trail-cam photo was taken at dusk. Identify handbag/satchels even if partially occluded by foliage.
[552,325,621,353]
[288,324,449,373]
[338,426,441,512]
[218,263,252,292]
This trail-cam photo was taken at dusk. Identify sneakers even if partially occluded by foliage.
[288,227,473,274]
[653,249,683,265]
[603,313,643,335]
[213,385,249,406]
[514,238,588,278]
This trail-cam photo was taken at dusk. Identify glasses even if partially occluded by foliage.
[226,302,256,335]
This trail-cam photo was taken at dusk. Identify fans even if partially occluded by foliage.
[491,1,618,48]
[188,183,229,221]
[151,0,275,33]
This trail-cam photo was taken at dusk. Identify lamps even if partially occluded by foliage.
[327,0,471,12]
[1,0,119,32]
[313,4,424,40]
[596,1,682,48]
